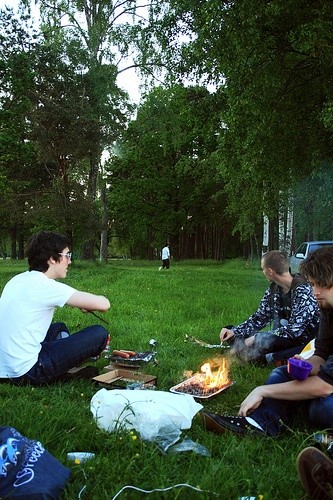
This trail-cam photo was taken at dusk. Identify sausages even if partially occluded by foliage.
[120,350,136,356]
[113,350,129,358]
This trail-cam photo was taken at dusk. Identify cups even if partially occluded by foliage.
[287,358,312,381]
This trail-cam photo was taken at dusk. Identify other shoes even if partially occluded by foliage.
[301,428,333,451]
[159,267,161,271]
[221,338,247,358]
[248,351,276,370]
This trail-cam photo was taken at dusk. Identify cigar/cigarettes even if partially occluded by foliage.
[219,341,223,348]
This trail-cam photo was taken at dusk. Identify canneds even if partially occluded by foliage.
[102,334,111,352]
[66,452,95,465]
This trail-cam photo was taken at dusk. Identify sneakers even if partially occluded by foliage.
[296,447,333,500]
[201,412,271,445]
[56,365,99,385]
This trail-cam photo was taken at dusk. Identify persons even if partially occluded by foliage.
[200,245,333,500]
[0,230,111,388]
[158,242,171,272]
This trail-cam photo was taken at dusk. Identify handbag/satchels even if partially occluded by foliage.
[0,426,72,500]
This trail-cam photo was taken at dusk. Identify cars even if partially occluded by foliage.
[288,240,333,277]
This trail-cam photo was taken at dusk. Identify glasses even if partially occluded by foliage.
[58,252,71,262]
